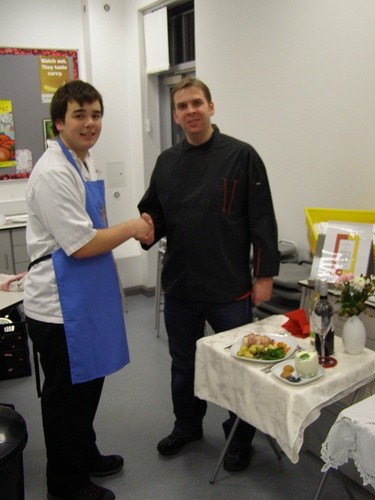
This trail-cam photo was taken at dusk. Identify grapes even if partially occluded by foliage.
[237,344,265,359]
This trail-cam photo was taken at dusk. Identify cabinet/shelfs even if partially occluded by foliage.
[0,227,32,275]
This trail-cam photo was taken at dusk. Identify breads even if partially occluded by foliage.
[281,365,294,379]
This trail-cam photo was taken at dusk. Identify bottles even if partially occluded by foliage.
[308,279,333,356]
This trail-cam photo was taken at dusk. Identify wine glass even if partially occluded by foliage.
[310,298,336,368]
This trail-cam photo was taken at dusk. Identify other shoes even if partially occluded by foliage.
[224,445,248,472]
[48,483,115,500]
[91,456,124,476]
[157,424,204,457]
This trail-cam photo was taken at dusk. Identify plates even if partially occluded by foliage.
[272,359,324,385]
[230,332,302,363]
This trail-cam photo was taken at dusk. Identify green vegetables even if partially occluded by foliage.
[253,346,286,360]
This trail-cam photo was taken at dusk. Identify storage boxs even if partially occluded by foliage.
[0,309,32,382]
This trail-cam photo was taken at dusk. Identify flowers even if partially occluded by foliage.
[331,273,375,317]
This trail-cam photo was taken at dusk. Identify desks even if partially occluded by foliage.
[0,290,42,398]
[313,394,375,500]
[298,279,375,351]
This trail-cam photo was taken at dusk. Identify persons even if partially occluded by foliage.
[135,78,283,471]
[25,79,153,500]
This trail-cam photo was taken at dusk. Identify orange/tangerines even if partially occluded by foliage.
[0,134,15,162]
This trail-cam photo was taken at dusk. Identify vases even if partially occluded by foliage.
[343,315,366,355]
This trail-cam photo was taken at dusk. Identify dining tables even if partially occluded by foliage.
[194,316,375,485]
